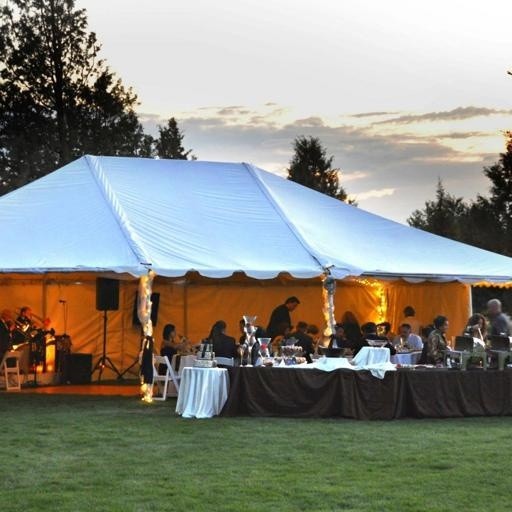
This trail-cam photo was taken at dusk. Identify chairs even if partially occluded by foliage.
[0,351,22,391]
[148,356,181,401]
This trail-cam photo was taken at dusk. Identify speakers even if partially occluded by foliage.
[55,351,92,385]
[95,277,119,310]
[132,290,160,327]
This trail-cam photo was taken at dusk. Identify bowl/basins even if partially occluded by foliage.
[364,339,389,347]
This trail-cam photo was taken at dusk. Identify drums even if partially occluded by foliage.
[56,336,71,349]
[40,331,56,345]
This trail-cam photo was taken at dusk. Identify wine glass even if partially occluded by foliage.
[236,314,271,368]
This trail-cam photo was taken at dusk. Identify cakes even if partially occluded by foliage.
[192,343,218,368]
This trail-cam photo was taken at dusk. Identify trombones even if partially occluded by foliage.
[13,306,50,327]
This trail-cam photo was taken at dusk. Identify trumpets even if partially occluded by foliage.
[11,317,29,332]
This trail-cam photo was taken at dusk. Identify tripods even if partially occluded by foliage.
[91,311,125,381]
[117,327,144,378]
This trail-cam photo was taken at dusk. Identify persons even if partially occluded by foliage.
[158,324,186,375]
[0,307,32,364]
[202,320,237,367]
[238,297,512,370]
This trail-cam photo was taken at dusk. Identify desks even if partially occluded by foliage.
[174,366,231,419]
[218,363,512,418]
